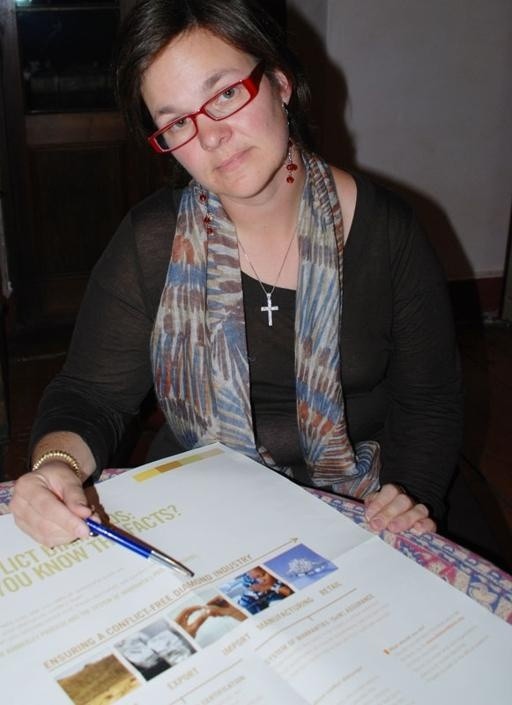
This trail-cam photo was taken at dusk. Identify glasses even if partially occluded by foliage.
[147,61,265,155]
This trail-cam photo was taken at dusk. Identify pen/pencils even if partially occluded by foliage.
[86,519,195,579]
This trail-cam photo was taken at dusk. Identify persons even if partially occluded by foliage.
[6,3,507,578]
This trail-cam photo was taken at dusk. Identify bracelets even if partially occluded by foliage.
[32,445,88,477]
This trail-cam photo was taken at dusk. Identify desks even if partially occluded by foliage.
[0,468,512,627]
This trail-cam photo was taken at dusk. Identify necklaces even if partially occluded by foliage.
[232,206,306,325]
